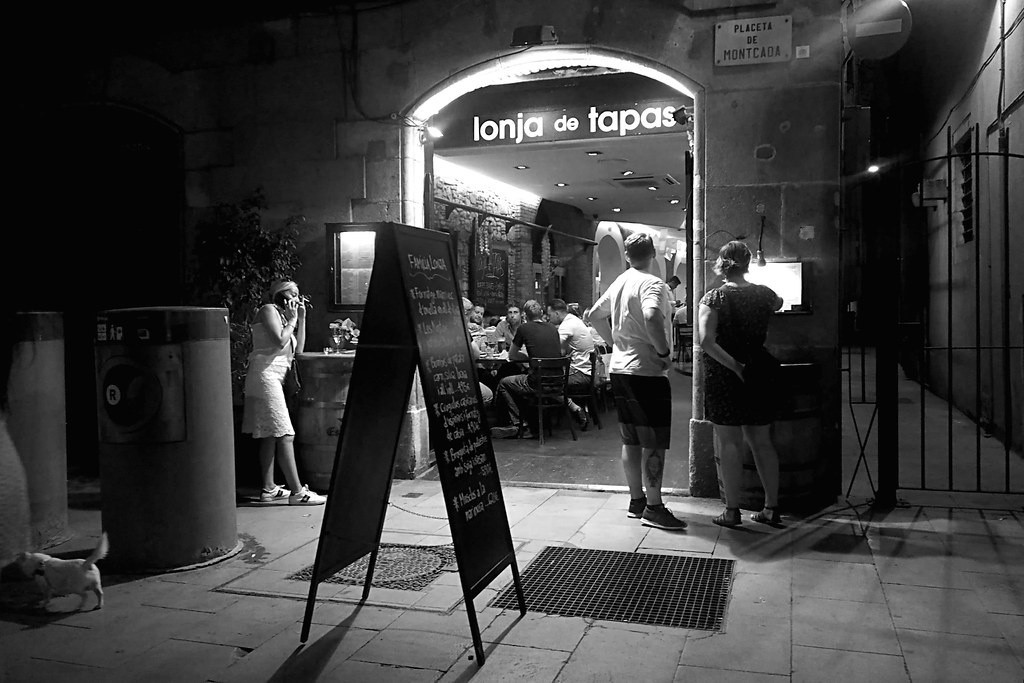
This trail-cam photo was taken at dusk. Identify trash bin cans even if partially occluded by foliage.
[4,310,72,551]
[92,303,245,572]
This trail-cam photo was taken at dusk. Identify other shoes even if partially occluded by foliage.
[576,405,590,430]
[519,427,534,439]
[490,426,518,439]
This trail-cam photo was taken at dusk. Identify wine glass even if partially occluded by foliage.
[333,334,343,354]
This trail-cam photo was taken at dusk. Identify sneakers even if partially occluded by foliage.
[289,484,327,505]
[260,484,292,502]
[641,505,687,529]
[627,498,647,518]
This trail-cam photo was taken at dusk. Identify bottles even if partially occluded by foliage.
[481,340,487,352]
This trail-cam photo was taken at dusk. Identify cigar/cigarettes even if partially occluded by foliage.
[299,295,310,302]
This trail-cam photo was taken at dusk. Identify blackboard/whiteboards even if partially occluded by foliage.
[312,221,516,599]
[469,246,508,308]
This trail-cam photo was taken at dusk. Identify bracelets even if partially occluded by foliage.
[288,324,296,328]
[657,349,671,358]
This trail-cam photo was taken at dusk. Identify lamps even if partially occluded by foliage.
[672,104,693,125]
[755,215,768,267]
[509,24,559,49]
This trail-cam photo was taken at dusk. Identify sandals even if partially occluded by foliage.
[751,507,782,524]
[712,507,742,525]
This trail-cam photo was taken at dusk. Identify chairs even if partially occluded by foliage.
[515,358,581,447]
[567,345,605,431]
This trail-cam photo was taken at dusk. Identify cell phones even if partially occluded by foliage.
[275,294,293,310]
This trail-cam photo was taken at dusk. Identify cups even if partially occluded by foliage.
[488,338,496,349]
[497,337,506,352]
[323,347,333,355]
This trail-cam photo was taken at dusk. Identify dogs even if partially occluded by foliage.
[19,532,110,612]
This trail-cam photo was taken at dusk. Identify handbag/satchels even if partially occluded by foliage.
[283,359,302,397]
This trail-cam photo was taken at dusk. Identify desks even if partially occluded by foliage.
[473,352,524,393]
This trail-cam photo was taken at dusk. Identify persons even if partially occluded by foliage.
[241,280,328,505]
[454,292,616,437]
[661,274,690,363]
[696,238,786,526]
[588,230,691,531]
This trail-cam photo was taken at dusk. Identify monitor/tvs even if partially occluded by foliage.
[743,260,807,313]
